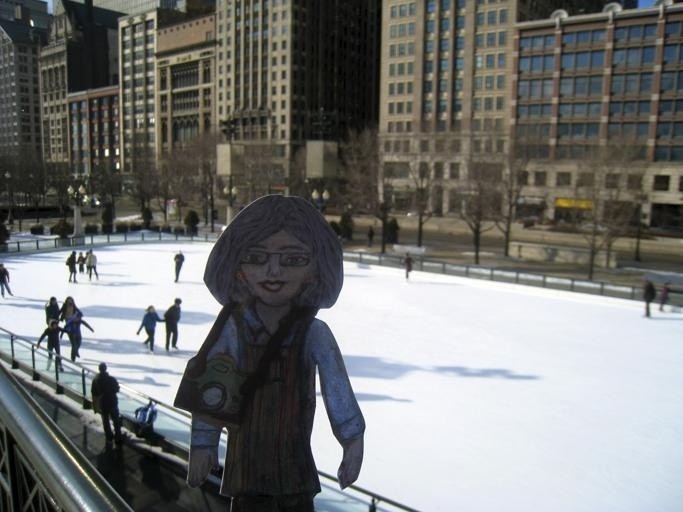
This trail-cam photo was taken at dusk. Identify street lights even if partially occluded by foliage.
[305,140,339,219]
[4,171,15,225]
[65,185,90,238]
[633,192,649,261]
[215,142,246,228]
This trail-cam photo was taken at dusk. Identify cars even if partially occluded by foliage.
[583,221,625,236]
[91,197,111,207]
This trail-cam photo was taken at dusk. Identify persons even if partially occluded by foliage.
[0,260,16,304]
[135,303,166,356]
[89,362,122,451]
[656,278,673,312]
[171,190,372,512]
[64,247,101,284]
[640,276,658,319]
[34,296,95,370]
[163,297,183,354]
[170,249,187,284]
[399,251,416,280]
[366,223,374,247]
